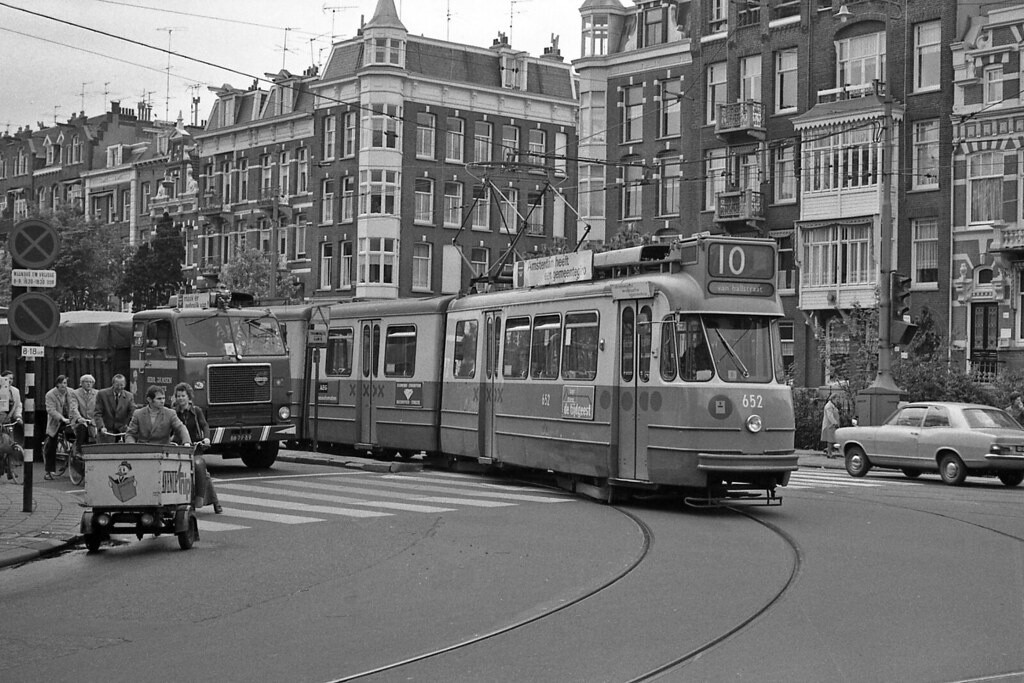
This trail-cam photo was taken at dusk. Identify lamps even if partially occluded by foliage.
[834,5,853,23]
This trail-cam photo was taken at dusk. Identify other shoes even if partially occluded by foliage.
[214,502,224,514]
[44,473,53,480]
[194,496,204,507]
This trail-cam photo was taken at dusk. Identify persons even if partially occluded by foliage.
[515,347,528,377]
[0,371,24,480]
[44,375,223,514]
[1004,391,1024,420]
[821,393,840,459]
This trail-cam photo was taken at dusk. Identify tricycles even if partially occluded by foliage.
[78,442,200,552]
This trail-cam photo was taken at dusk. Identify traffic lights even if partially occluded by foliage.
[889,273,919,347]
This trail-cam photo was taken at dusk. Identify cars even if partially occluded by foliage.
[834,402,1023,489]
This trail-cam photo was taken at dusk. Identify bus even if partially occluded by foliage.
[268,152,799,512]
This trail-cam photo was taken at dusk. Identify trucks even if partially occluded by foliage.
[0,285,298,468]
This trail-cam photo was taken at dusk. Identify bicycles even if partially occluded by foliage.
[0,420,25,485]
[170,440,216,506]
[102,429,127,442]
[41,419,92,486]
